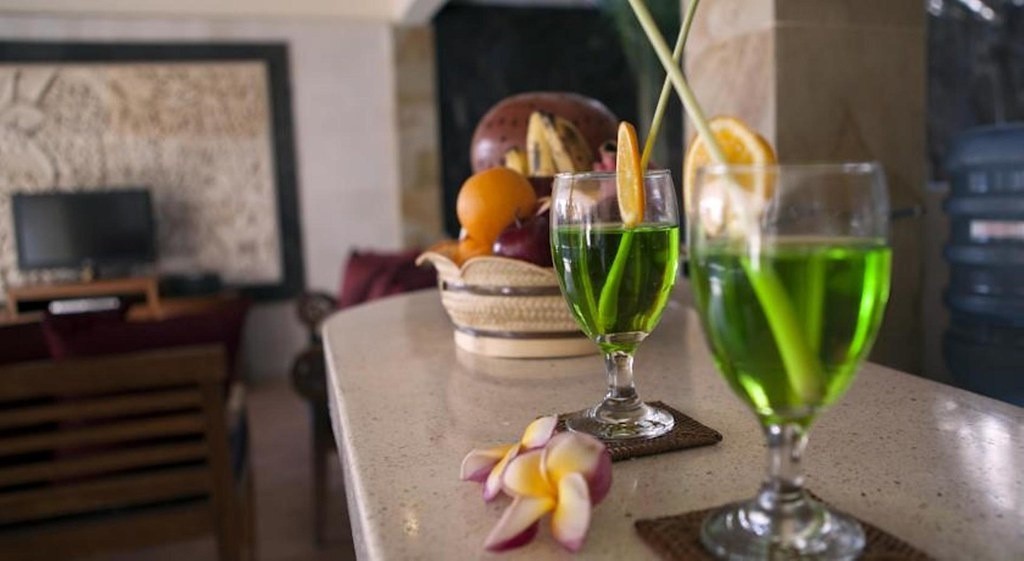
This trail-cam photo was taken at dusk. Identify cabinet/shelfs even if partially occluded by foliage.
[5,275,164,322]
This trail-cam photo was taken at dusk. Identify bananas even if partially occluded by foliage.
[508,107,599,177]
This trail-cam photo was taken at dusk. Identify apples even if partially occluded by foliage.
[492,213,551,268]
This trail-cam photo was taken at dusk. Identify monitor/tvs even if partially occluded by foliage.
[12,187,157,285]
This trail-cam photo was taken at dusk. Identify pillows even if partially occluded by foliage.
[41,291,253,493]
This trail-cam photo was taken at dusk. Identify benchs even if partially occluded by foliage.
[0,341,259,561]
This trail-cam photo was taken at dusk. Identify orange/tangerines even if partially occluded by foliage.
[680,116,778,227]
[614,121,645,226]
[457,167,536,262]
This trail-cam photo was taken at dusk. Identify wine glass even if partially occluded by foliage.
[686,159,892,561]
[550,170,680,442]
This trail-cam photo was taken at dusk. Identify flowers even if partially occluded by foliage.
[462,410,614,553]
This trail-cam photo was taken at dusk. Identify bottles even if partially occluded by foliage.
[937,122,1023,397]
